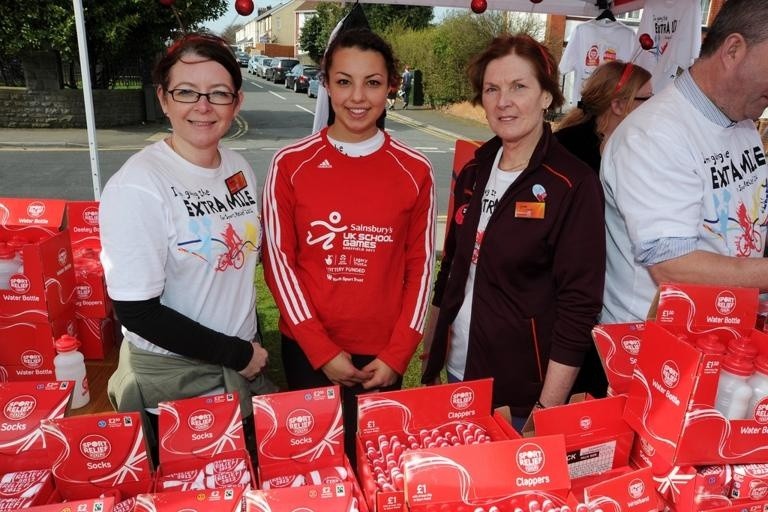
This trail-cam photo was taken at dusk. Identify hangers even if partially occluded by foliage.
[595,0,617,21]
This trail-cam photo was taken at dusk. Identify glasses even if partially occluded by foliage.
[162,87,238,105]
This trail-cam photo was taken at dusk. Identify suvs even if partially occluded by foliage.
[266,58,300,84]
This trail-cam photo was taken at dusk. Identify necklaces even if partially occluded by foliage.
[497,147,527,171]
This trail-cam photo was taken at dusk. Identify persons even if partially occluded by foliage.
[261,28,437,422]
[599,0,768,328]
[418,30,609,436]
[97,33,269,434]
[551,61,654,176]
[385,65,413,110]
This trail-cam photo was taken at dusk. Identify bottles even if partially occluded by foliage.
[714,337,757,422]
[53,335,91,409]
[746,354,768,422]
[0,245,19,289]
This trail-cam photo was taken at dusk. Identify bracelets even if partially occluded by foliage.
[535,400,546,410]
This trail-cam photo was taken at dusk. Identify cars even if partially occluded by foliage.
[308,71,320,97]
[235,52,251,66]
[248,56,268,75]
[285,65,320,92]
[256,58,273,79]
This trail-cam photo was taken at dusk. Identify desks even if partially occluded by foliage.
[63,348,121,418]
